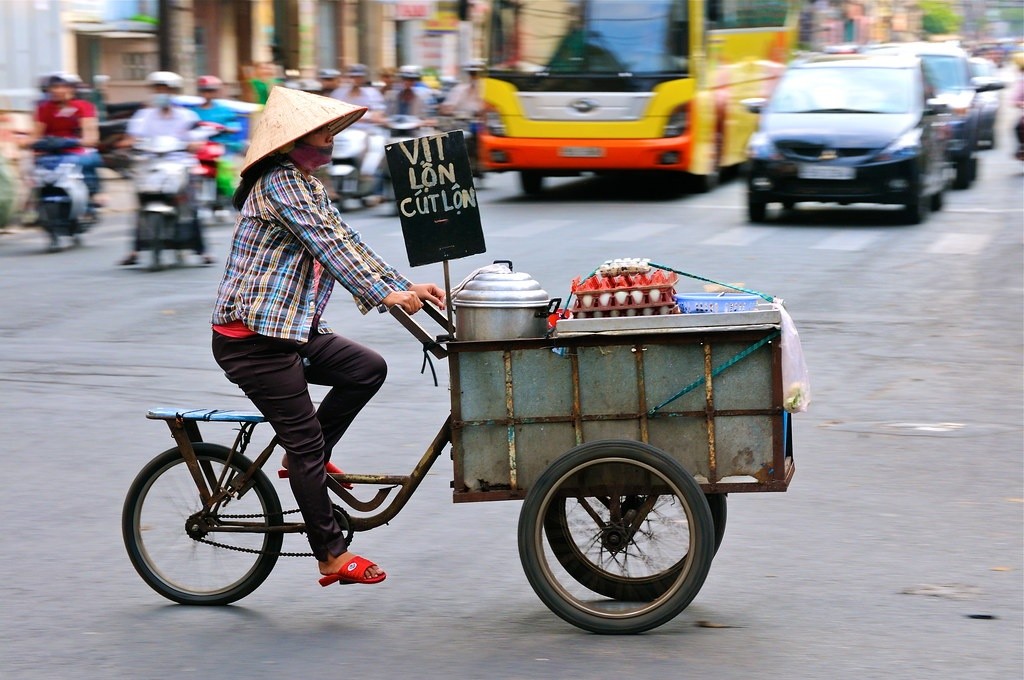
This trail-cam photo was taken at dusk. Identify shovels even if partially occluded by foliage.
[689,292,725,313]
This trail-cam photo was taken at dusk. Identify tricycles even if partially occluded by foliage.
[120,293,798,634]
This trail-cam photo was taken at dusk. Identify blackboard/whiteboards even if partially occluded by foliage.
[385,130,487,268]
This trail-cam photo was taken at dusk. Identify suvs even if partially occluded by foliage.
[742,35,1009,224]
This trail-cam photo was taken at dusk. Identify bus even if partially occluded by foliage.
[475,1,802,199]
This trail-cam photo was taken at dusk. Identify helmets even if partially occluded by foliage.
[37,72,58,87]
[144,70,183,89]
[318,69,341,79]
[345,63,369,78]
[464,61,485,73]
[196,76,221,90]
[397,65,421,78]
[50,72,81,88]
[301,80,321,91]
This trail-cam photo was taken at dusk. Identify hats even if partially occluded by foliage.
[239,85,369,177]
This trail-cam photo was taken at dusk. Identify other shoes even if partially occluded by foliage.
[192,250,215,264]
[119,252,138,266]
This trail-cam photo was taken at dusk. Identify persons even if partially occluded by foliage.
[1008,52,1023,168]
[283,59,499,210]
[115,71,242,270]
[18,70,101,248]
[210,85,445,587]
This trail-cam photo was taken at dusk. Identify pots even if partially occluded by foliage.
[453,260,560,342]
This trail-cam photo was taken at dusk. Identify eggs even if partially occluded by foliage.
[576,289,671,318]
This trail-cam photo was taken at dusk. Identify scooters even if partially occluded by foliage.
[330,116,441,209]
[19,137,105,251]
[130,120,243,273]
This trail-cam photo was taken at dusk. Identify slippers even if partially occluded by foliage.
[318,555,387,587]
[278,460,354,489]
